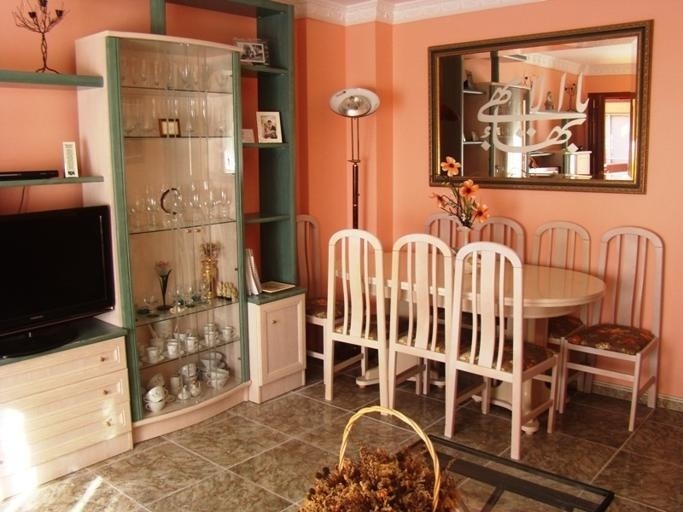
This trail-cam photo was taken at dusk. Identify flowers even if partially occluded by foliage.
[298,443,468,512]
[10,0,70,74]
[425,155,490,229]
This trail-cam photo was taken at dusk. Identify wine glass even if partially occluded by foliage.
[115,43,229,138]
[123,182,235,231]
[140,266,211,318]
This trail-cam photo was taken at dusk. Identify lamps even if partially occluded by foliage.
[328,87,381,229]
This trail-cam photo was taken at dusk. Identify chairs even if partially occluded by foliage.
[458,216,526,388]
[387,233,483,423]
[444,241,561,461]
[325,228,423,417]
[504,220,592,410]
[557,226,665,434]
[296,214,369,384]
[414,212,470,370]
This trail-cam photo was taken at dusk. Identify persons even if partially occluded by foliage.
[264,120,277,139]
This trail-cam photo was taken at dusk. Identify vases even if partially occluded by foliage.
[454,226,480,274]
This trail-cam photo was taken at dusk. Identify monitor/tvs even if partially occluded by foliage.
[0,205,115,358]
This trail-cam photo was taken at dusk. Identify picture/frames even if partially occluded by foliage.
[158,119,182,138]
[255,111,283,143]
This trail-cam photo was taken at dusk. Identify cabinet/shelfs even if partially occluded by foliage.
[150,0,296,291]
[246,281,307,404]
[0,317,135,505]
[440,53,487,181]
[74,29,250,444]
[0,69,104,188]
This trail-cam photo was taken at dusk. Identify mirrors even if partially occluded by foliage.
[426,20,654,195]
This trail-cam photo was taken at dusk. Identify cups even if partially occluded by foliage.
[136,320,238,413]
